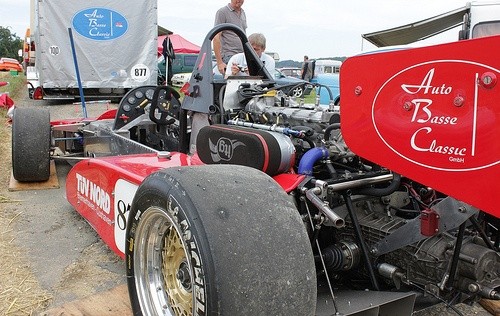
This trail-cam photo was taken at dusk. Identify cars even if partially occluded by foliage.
[0,58,23,73]
[275,69,313,97]
[171,73,193,89]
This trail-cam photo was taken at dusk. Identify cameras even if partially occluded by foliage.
[236,65,248,73]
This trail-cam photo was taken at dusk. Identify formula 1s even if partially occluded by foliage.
[10,23,499,316]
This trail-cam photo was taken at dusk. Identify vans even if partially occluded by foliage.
[158,53,200,81]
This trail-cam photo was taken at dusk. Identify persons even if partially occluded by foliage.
[224,32,277,78]
[213,0,248,78]
[302,55,312,81]
